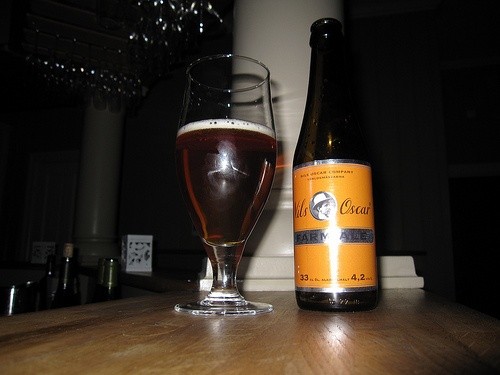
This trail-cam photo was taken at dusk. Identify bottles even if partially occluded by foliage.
[291,16,383,316]
[4,252,120,318]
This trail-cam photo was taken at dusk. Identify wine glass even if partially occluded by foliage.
[173,54,279,319]
